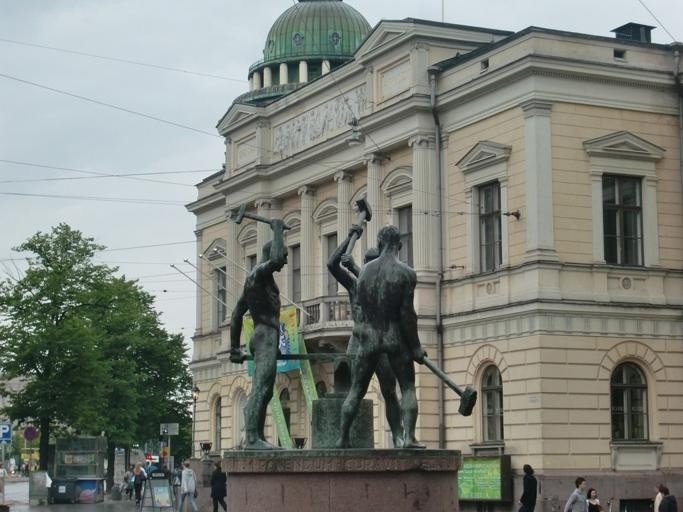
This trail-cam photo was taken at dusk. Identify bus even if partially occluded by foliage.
[20,448,40,460]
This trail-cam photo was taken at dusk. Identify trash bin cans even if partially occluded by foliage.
[542,495,560,512]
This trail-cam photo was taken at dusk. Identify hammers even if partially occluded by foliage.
[422,354,477,417]
[235,203,292,230]
[344,195,372,262]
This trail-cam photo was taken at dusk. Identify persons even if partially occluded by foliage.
[657,485,678,512]
[131,460,147,504]
[122,465,134,499]
[652,482,664,511]
[227,202,292,451]
[335,224,478,448]
[208,461,227,511]
[326,195,405,448]
[564,476,587,511]
[517,464,537,512]
[0,463,6,505]
[585,487,603,511]
[178,459,198,511]
